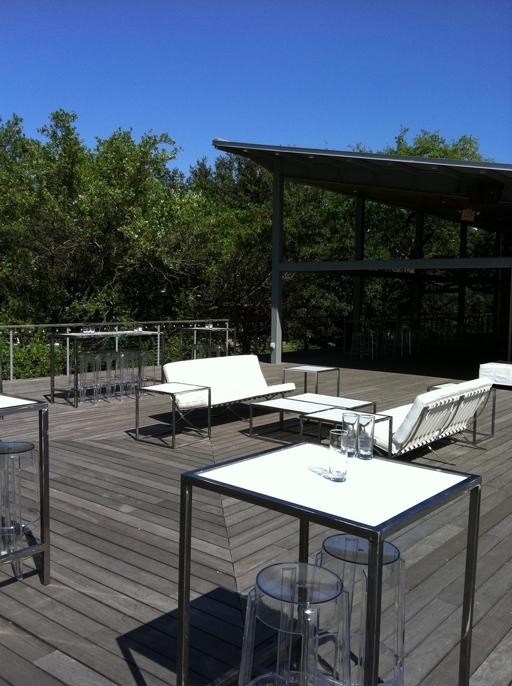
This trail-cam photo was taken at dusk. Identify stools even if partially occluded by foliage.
[0,441,37,581]
[238,533,407,686]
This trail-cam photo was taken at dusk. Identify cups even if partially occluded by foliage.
[82,326,95,333]
[328,427,348,483]
[205,318,213,328]
[358,414,374,459]
[342,411,359,457]
[133,320,142,331]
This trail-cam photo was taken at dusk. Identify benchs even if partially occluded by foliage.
[362,378,493,458]
[163,354,296,437]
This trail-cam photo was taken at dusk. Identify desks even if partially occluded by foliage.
[177,442,482,686]
[135,382,211,449]
[281,365,339,399]
[0,392,51,585]
[299,407,392,459]
[427,382,496,445]
